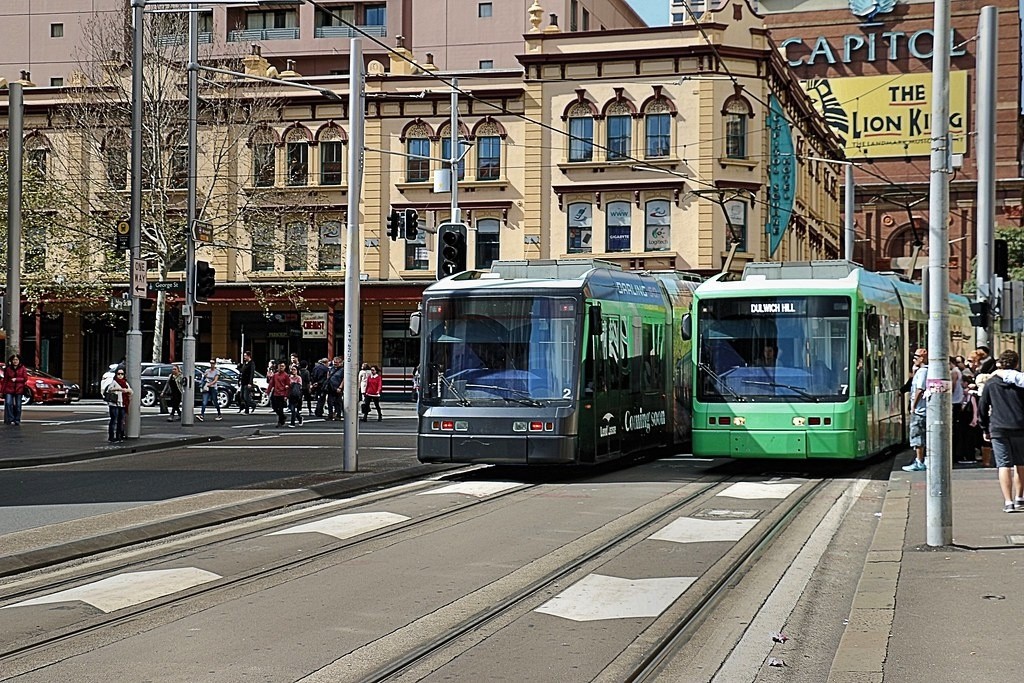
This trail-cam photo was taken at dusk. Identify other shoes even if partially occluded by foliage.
[108,439,120,444]
[215,415,223,421]
[116,438,123,442]
[176,416,181,420]
[306,411,341,421]
[360,418,367,422]
[378,416,382,421]
[167,417,173,422]
[243,413,249,415]
[7,420,20,425]
[298,417,304,426]
[288,424,295,427]
[196,415,204,421]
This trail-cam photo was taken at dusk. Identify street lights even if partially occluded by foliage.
[182,61,341,426]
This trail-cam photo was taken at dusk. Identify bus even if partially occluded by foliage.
[681,258,979,468]
[410,259,710,468]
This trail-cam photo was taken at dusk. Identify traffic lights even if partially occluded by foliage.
[194,260,215,304]
[436,223,468,280]
[969,300,988,331]
[405,208,419,240]
[387,209,399,241]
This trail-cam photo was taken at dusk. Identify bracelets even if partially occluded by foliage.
[338,388,342,389]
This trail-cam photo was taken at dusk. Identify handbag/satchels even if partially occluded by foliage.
[361,404,371,413]
[104,393,118,402]
[24,385,32,398]
[321,380,330,393]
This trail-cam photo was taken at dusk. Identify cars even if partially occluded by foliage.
[100,360,261,407]
[170,361,272,407]
[0,362,81,405]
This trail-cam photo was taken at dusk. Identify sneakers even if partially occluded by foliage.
[1003,504,1014,512]
[1014,500,1024,511]
[901,461,926,471]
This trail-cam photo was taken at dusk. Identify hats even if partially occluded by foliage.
[910,349,927,358]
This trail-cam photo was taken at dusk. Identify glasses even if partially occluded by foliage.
[117,373,124,375]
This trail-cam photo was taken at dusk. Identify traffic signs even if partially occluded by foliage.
[133,258,147,298]
[190,218,214,244]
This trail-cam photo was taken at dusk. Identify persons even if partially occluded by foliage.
[265,359,304,427]
[751,344,784,367]
[108,366,133,443]
[195,358,223,421]
[288,353,301,375]
[299,360,315,416]
[236,351,256,414]
[0,354,28,425]
[160,365,184,422]
[979,349,1024,513]
[311,355,344,421]
[949,346,1000,463]
[359,362,371,402]
[359,365,383,422]
[902,348,928,472]
